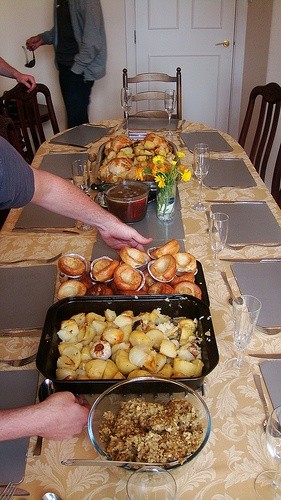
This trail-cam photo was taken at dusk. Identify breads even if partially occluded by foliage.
[58,239,201,301]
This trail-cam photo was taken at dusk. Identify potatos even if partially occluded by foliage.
[55,309,204,379]
[103,140,170,166]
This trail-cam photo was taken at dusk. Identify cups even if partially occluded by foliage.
[126,466,177,500]
[72,159,90,192]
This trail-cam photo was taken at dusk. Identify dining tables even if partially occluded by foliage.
[0,116,281,500]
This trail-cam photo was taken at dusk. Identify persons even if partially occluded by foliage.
[0,136,154,441]
[0,57,36,93]
[26,0,106,129]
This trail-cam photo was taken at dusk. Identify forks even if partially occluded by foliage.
[0,353,37,367]
[0,253,62,265]
[0,482,17,499]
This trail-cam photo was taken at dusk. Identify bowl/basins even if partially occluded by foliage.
[88,376,211,472]
[105,180,150,223]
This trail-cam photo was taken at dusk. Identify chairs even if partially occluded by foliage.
[122,67,183,131]
[238,82,281,184]
[0,80,61,166]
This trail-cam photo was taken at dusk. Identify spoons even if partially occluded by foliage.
[254,375,281,438]
[33,378,55,456]
[42,492,61,500]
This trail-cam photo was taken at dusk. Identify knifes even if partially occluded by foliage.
[0,486,31,496]
[11,228,79,236]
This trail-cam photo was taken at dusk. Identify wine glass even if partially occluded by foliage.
[207,212,229,268]
[164,89,176,138]
[193,143,210,211]
[255,405,281,500]
[120,87,132,137]
[225,294,262,375]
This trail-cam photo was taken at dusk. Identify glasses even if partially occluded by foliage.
[21,45,35,68]
